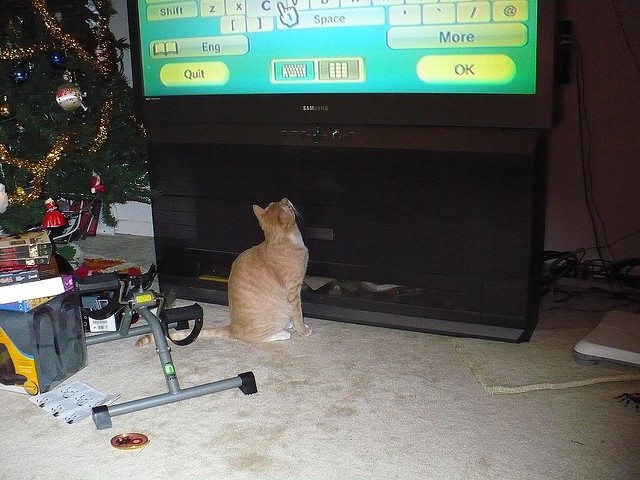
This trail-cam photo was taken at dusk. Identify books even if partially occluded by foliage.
[0,229,75,314]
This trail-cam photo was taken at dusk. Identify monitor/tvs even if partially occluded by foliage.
[127,0,555,130]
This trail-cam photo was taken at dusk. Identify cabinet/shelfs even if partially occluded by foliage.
[141,124,540,345]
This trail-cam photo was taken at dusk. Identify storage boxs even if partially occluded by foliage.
[0,288,88,397]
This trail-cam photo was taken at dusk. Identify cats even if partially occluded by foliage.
[133,197,315,349]
